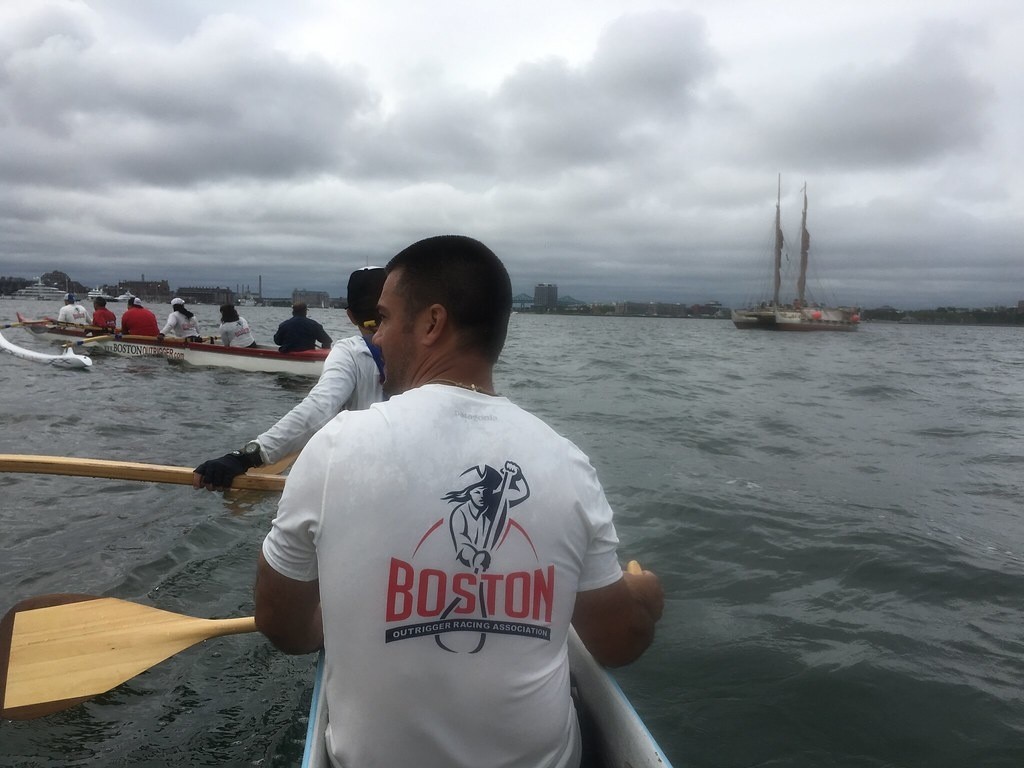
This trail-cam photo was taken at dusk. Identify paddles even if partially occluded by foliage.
[52,320,122,333]
[315,344,321,349]
[186,335,221,343]
[249,452,300,475]
[2,557,644,713]
[61,335,108,348]
[0,320,49,329]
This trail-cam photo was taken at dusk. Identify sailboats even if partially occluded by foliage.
[729,172,862,332]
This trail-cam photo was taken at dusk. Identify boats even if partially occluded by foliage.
[0,310,332,377]
[11,283,81,301]
[899,316,919,324]
[238,295,256,306]
[302,622,677,768]
[87,289,119,302]
[114,291,136,301]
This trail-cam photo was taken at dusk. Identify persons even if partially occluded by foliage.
[794,299,800,309]
[274,302,333,350]
[193,268,390,492]
[58,293,93,325]
[92,297,116,336]
[158,297,200,342]
[253,235,663,768]
[122,297,159,336]
[219,305,258,348]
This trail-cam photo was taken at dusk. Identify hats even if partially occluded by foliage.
[126,297,142,307]
[293,302,310,312]
[64,293,77,302]
[170,297,183,306]
[345,267,386,309]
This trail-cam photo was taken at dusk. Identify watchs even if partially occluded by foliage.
[244,442,263,465]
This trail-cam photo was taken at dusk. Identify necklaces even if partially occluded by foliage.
[426,379,499,396]
